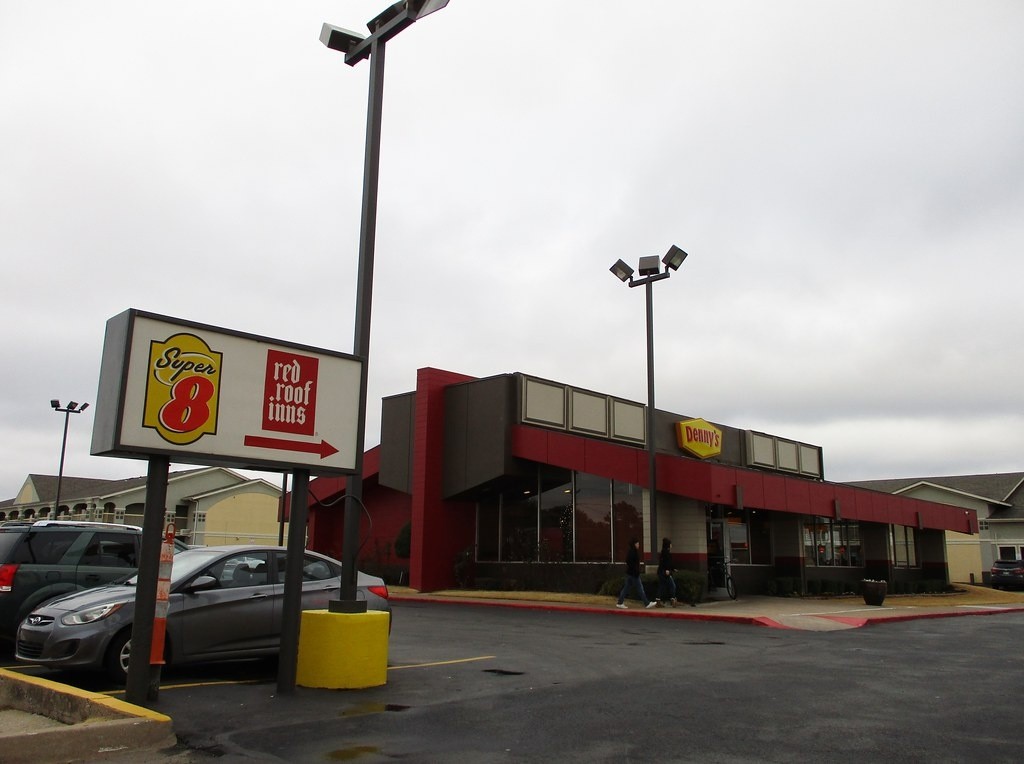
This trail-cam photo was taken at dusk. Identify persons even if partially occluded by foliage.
[655,536,678,607]
[617,538,657,609]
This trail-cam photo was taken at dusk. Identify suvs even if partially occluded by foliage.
[0,523,191,646]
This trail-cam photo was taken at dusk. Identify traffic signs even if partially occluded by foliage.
[92,308,364,477]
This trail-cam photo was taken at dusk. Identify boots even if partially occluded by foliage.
[671,598,678,608]
[656,599,666,607]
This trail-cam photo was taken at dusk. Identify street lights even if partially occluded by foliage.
[50,400,89,520]
[610,245,688,563]
[319,0,449,614]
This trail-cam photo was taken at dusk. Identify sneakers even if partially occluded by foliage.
[616,603,629,609]
[646,601,657,609]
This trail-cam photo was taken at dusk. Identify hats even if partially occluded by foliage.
[662,537,671,547]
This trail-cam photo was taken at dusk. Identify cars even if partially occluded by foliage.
[16,544,393,684]
[33,521,142,530]
[990,560,1024,590]
